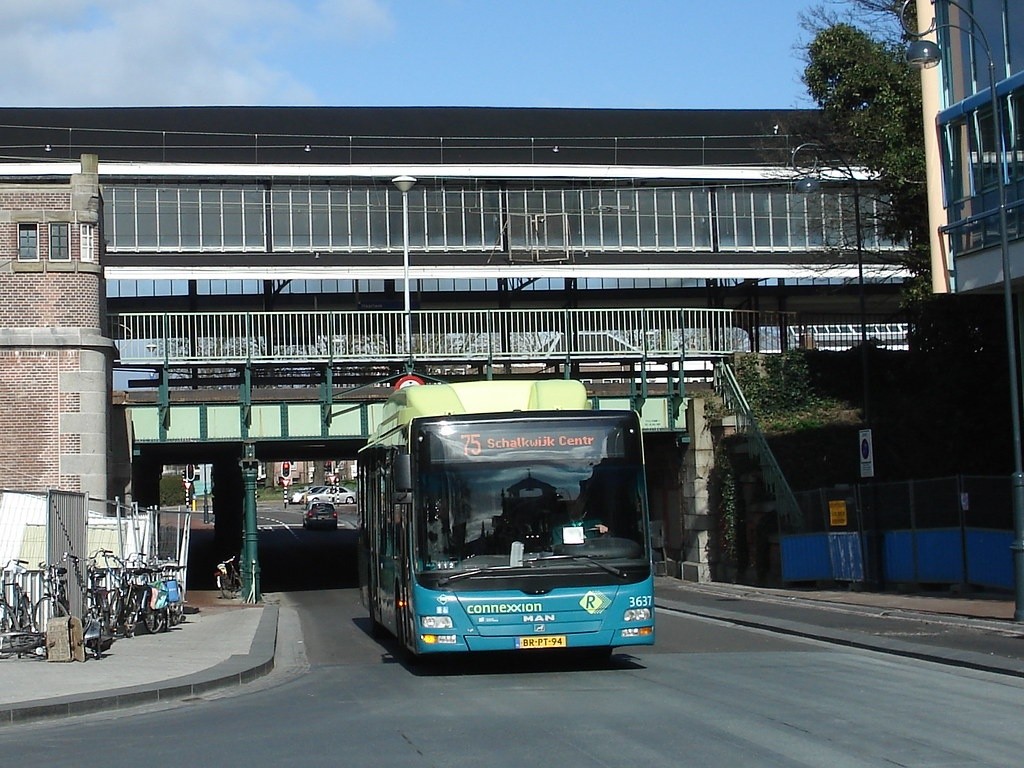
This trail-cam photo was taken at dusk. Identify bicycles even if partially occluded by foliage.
[214,555,241,599]
[0,549,186,662]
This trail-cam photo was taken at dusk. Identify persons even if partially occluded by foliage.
[552,500,609,546]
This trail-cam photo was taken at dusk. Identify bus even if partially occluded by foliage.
[355,377,656,666]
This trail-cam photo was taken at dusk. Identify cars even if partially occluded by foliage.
[301,501,338,530]
[305,485,357,505]
[288,484,325,506]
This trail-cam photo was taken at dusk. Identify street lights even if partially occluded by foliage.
[898,0,1024,624]
[391,173,424,387]
[790,142,880,596]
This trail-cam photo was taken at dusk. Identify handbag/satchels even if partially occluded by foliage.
[151,581,169,609]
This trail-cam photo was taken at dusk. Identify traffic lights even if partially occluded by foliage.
[187,464,194,480]
[283,463,290,477]
